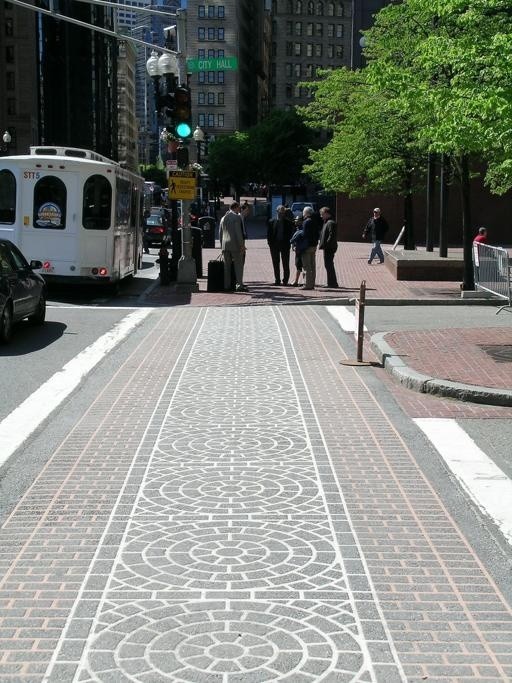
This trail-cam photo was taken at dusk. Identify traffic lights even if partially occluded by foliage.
[173,86,192,141]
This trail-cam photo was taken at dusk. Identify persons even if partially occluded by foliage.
[159,207,171,225]
[289,214,305,286]
[317,206,339,287]
[219,202,249,292]
[266,204,295,286]
[142,207,151,253]
[471,226,492,282]
[190,198,249,245]
[297,205,320,290]
[160,191,168,202]
[361,207,390,265]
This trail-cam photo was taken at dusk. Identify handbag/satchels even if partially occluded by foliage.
[206,252,238,293]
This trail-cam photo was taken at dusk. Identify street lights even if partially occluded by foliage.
[194,124,204,216]
[145,23,193,226]
[2,131,12,155]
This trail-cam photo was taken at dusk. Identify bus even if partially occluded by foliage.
[0,145,146,298]
[144,181,165,207]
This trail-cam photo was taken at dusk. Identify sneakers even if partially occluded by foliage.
[275,281,339,289]
[367,259,384,263]
[235,284,249,292]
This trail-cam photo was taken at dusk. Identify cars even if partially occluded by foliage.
[0,238,48,345]
[145,207,181,245]
[291,202,320,219]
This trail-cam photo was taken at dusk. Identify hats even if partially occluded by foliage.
[373,207,380,212]
